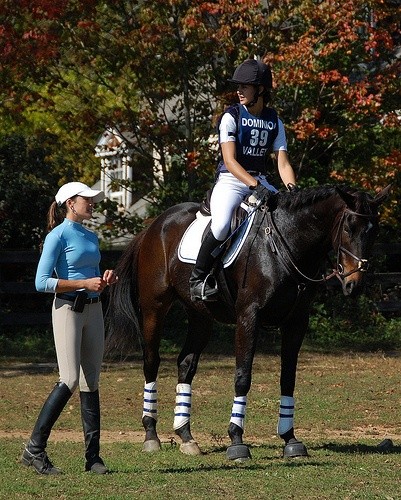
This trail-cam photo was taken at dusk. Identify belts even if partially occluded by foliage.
[56,293,102,304]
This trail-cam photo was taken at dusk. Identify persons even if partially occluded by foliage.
[21,181,118,476]
[189,60,296,299]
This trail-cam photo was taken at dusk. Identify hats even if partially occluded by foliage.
[55,181,106,207]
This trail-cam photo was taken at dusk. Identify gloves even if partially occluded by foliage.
[249,179,277,212]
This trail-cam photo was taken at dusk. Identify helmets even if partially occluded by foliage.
[228,60,273,88]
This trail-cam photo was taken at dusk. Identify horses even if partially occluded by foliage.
[102,182,393,461]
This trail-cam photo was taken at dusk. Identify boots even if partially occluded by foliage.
[79,388,108,474]
[21,381,73,474]
[189,229,221,302]
